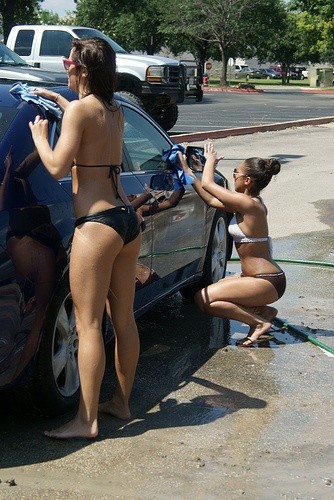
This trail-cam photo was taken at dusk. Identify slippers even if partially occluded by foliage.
[236,335,274,347]
[266,324,287,333]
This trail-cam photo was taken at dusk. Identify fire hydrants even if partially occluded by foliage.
[203,73,210,88]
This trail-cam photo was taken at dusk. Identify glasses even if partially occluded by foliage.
[63,60,83,70]
[233,168,248,178]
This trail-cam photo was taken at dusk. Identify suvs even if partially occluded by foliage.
[0,24,204,132]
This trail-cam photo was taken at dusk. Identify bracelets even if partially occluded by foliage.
[54,94,60,102]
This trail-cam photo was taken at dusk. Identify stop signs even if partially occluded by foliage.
[206,62,212,70]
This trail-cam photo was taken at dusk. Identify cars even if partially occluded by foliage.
[0,78,235,411]
[0,42,68,84]
[231,64,308,80]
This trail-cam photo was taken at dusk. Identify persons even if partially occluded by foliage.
[178,142,287,344]
[126,184,186,229]
[29,36,142,438]
[0,148,68,383]
